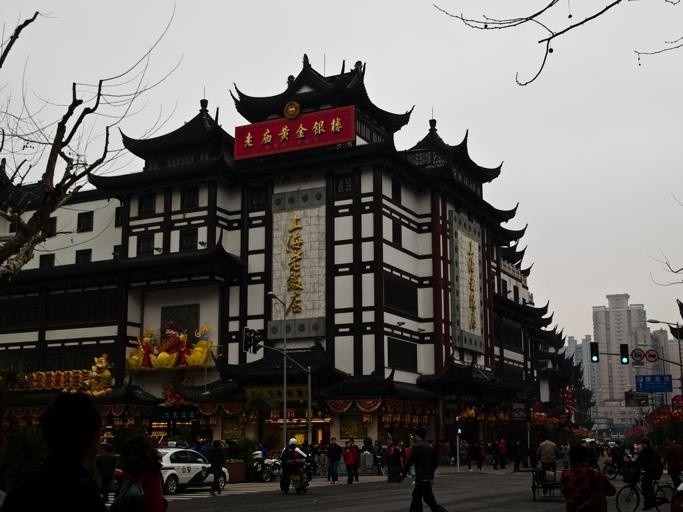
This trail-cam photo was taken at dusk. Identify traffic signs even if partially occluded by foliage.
[634,374,671,393]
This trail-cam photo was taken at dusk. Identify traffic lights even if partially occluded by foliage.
[254,332,262,352]
[590,342,598,361]
[456,422,462,434]
[620,344,628,363]
[245,327,254,349]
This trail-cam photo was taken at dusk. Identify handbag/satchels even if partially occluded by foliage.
[108,478,146,512]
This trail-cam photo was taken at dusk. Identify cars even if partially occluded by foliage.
[156,440,229,495]
[589,437,642,457]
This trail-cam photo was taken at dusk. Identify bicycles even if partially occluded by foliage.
[615,465,674,512]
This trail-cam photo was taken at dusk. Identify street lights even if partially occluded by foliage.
[647,316,683,389]
[268,291,287,447]
[636,342,668,404]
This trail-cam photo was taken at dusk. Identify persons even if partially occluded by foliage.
[256,433,411,492]
[0,390,226,512]
[137,327,156,368]
[401,428,447,512]
[437,426,682,511]
[154,320,190,367]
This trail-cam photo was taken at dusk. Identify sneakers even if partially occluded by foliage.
[642,506,652,511]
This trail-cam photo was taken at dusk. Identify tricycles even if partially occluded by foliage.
[527,454,569,501]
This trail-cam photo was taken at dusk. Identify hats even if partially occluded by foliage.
[638,438,650,444]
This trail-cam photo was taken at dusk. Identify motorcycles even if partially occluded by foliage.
[602,451,636,480]
[286,451,312,494]
[251,450,282,482]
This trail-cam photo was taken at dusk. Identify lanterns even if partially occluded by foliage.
[529,410,569,428]
[653,408,683,426]
